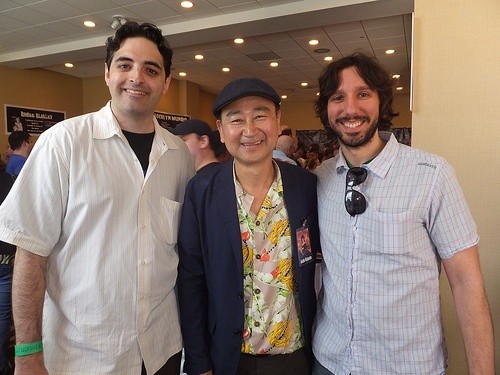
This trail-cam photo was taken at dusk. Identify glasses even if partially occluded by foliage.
[344,167,368,216]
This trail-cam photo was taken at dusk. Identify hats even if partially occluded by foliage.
[172,120,211,137]
[212,79,281,119]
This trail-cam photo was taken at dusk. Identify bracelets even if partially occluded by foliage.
[14,340,43,357]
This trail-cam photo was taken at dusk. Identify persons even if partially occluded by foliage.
[0,130,34,375]
[0,21,197,375]
[170,119,340,173]
[312,51,494,375]
[176,78,319,375]
